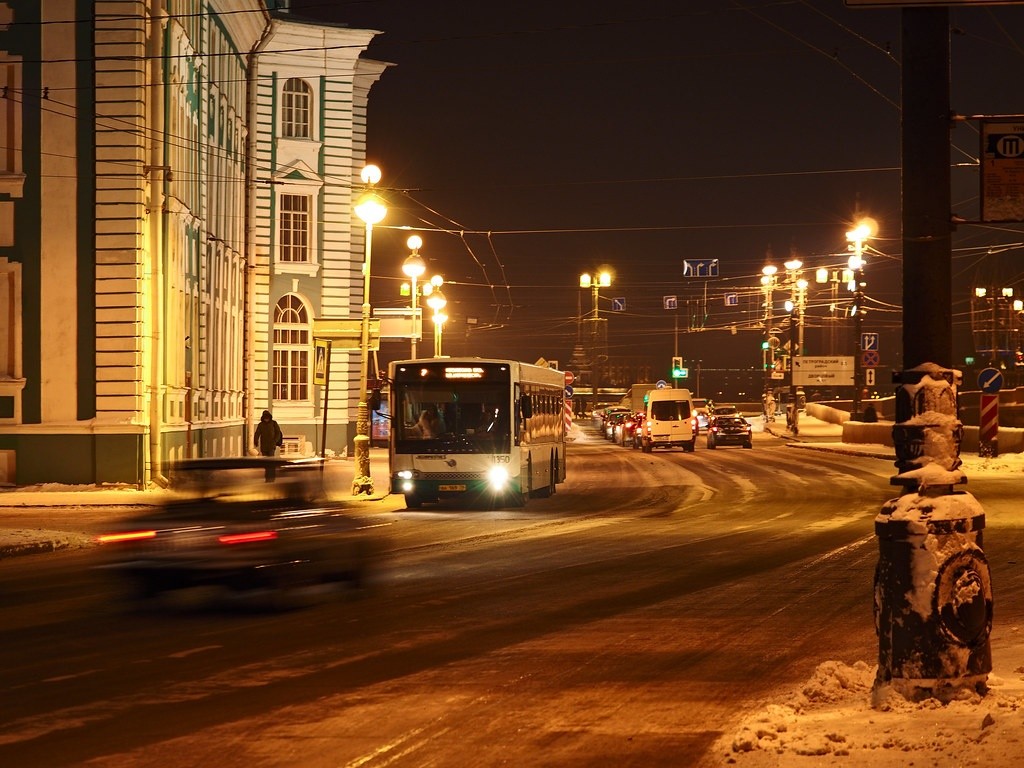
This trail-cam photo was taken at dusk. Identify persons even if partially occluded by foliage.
[253,410,283,484]
[862,403,878,422]
[403,401,497,440]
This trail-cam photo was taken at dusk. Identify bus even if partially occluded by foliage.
[370,356,566,510]
[571,388,629,413]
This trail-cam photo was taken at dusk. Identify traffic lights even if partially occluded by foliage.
[672,356,689,379]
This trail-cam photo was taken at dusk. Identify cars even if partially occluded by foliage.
[591,403,672,450]
[100,451,389,611]
[705,405,753,450]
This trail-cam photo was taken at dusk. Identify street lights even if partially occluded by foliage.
[849,225,871,422]
[975,282,1023,366]
[760,259,854,417]
[353,165,389,498]
[402,236,426,362]
[423,277,447,358]
[579,271,611,411]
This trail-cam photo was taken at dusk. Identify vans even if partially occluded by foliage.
[641,388,699,453]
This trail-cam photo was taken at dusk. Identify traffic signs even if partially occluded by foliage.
[792,356,855,387]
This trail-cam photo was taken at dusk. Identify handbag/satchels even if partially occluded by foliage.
[276,431,282,446]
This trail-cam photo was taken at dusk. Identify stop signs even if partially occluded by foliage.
[563,371,575,386]
